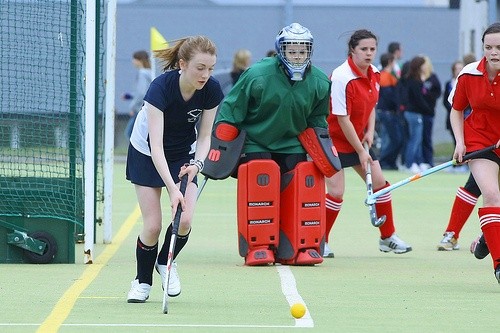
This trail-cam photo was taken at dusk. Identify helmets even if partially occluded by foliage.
[276,23,314,81]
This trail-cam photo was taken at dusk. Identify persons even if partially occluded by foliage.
[323,28,412,259]
[200,22,340,266]
[438,22,500,283]
[123,34,224,302]
[374,41,442,174]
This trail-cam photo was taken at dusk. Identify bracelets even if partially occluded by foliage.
[190,159,204,174]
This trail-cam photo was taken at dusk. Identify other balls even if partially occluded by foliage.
[290,303,306,318]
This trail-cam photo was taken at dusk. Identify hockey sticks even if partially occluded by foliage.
[363,140,387,227]
[364,145,497,205]
[162,163,190,314]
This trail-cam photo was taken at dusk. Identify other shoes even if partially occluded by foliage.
[410,163,421,173]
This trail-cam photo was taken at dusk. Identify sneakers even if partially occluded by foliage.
[127,273,153,303]
[323,242,334,258]
[470,232,490,259]
[379,232,412,254]
[436,232,459,250]
[155,258,181,297]
